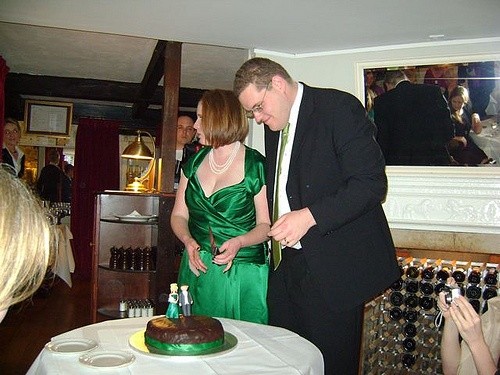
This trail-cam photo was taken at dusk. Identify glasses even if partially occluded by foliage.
[247,80,272,117]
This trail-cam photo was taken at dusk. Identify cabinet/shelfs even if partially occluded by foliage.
[90,189,160,324]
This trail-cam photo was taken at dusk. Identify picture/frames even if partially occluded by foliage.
[119,135,156,191]
[24,100,73,138]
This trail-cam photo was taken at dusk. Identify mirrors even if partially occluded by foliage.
[356,53,500,174]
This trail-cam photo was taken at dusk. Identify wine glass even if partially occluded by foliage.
[490,118,497,136]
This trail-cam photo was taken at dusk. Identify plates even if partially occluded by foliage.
[47,338,97,353]
[78,350,135,368]
[113,214,153,223]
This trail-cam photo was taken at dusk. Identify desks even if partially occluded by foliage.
[50,224,75,289]
[465,116,500,166]
[25,315,324,375]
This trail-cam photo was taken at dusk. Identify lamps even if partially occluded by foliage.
[120,130,156,194]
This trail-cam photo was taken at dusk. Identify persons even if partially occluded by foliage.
[172,115,203,195]
[0,119,26,182]
[438,263,500,375]
[230,57,400,375]
[178,285,195,316]
[0,161,57,324]
[363,60,499,168]
[169,88,274,330]
[166,283,179,319]
[284,238,289,244]
[35,149,73,206]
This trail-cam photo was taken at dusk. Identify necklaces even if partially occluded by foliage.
[208,140,240,175]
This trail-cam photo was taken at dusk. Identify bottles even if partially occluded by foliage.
[109,244,157,272]
[362,255,500,374]
[119,295,154,318]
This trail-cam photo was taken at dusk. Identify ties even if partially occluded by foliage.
[271,122,290,270]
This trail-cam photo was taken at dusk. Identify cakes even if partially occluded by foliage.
[144,283,224,352]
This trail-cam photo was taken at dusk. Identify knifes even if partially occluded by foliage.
[208,226,222,266]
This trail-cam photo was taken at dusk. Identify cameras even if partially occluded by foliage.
[445,288,461,307]
